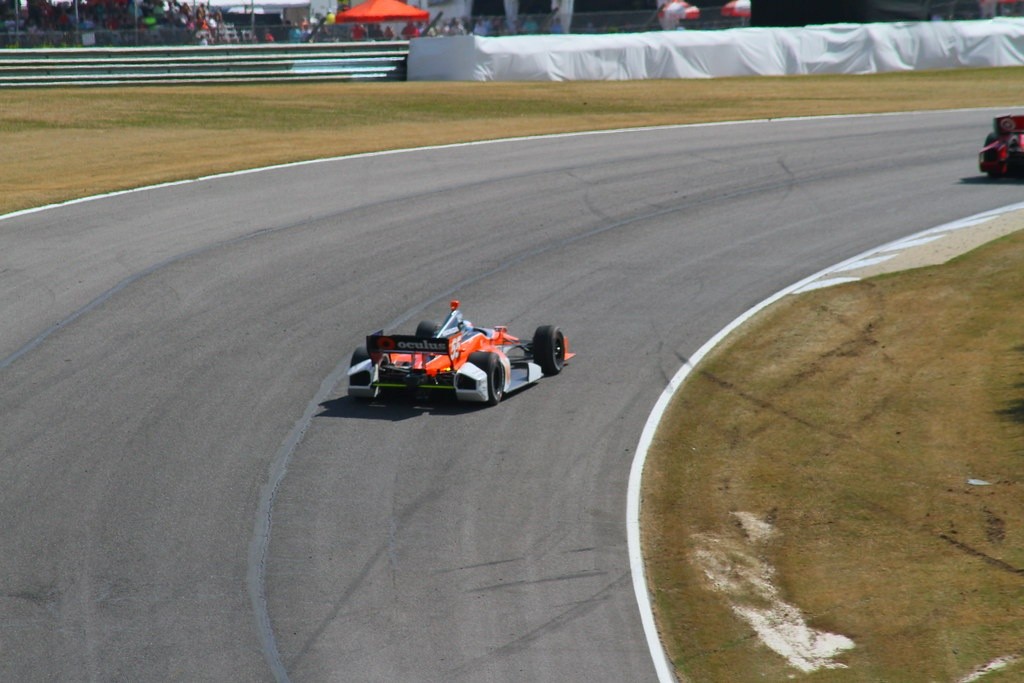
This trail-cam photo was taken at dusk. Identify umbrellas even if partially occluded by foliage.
[659,0,699,19]
[721,0,751,25]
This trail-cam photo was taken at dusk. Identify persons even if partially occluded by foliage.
[607,22,618,33]
[0,0,222,45]
[402,15,537,40]
[265,28,274,42]
[551,17,564,34]
[285,16,310,43]
[353,24,364,40]
[374,24,394,41]
[326,11,335,24]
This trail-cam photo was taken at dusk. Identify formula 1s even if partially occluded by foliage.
[345,301,578,407]
[978,115,1024,180]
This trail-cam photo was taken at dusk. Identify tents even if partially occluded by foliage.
[336,0,429,22]
